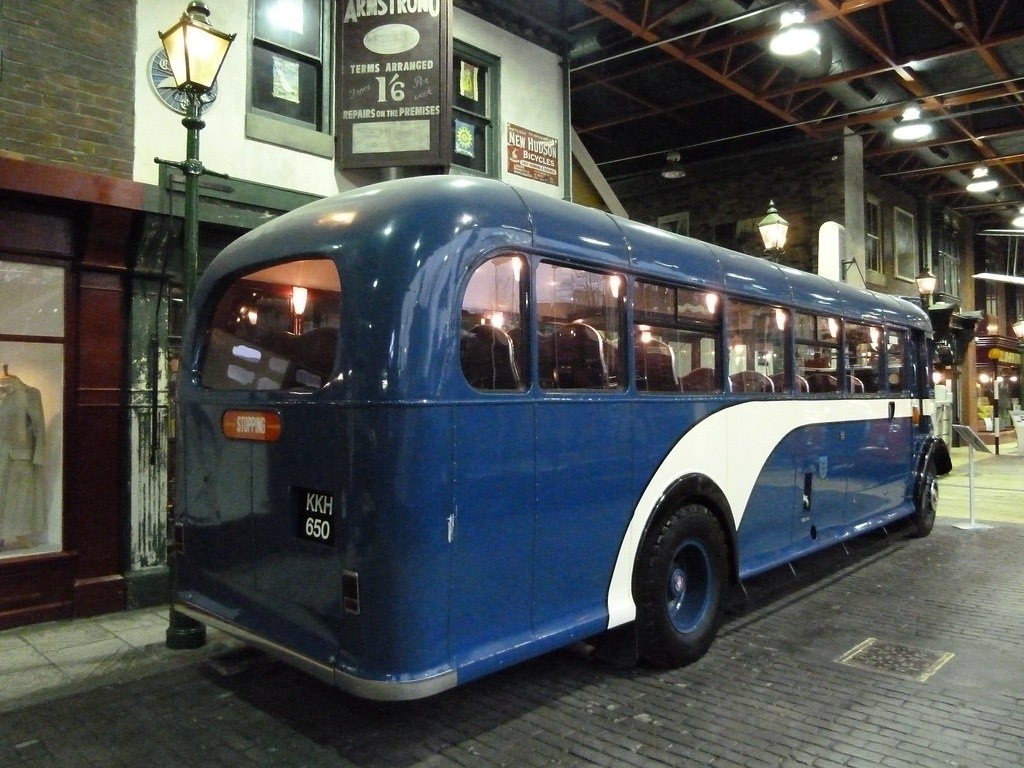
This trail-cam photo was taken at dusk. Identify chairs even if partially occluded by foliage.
[677,367,732,393]
[462,325,521,389]
[847,374,865,393]
[770,373,810,392]
[730,370,775,393]
[805,374,837,392]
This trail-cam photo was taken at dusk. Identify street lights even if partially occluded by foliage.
[757,198,790,263]
[1011,314,1024,414]
[914,264,937,316]
[156,0,237,649]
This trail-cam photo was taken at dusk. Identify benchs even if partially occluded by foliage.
[604,338,679,390]
[507,324,610,387]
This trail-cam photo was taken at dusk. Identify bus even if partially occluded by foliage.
[170,171,955,705]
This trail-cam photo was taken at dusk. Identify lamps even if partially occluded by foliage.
[771,9,821,58]
[966,162,998,193]
[892,101,931,141]
